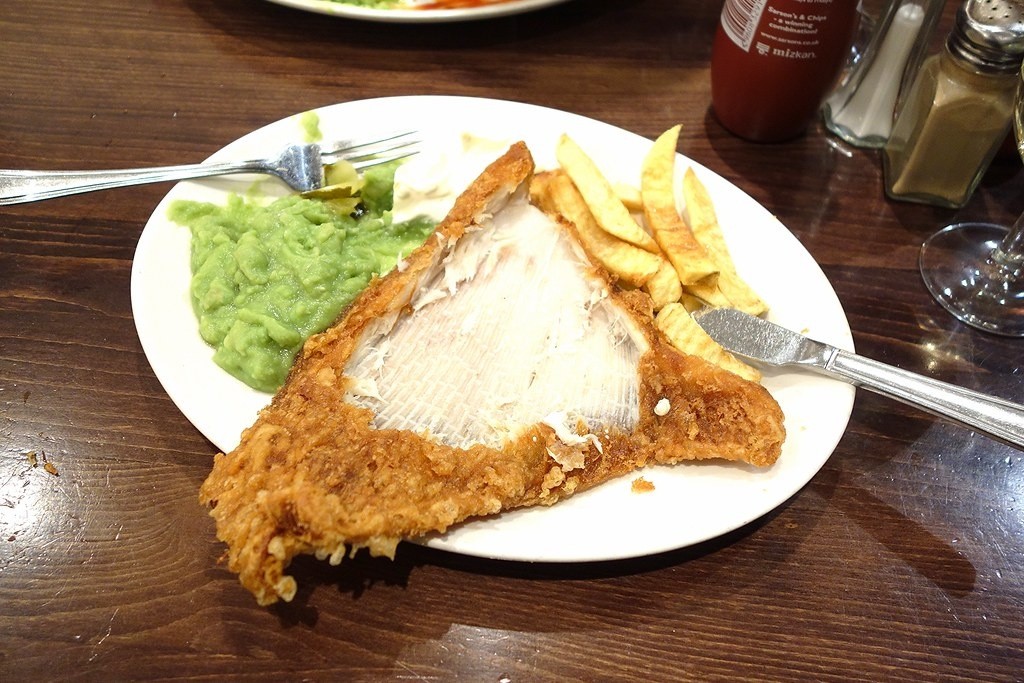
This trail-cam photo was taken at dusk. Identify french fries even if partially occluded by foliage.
[535,123,768,382]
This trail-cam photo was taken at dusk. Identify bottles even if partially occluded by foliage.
[820,0,942,149]
[714,1,862,143]
[883,1,1024,209]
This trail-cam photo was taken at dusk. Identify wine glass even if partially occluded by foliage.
[920,212,1024,336]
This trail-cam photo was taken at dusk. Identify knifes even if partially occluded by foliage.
[683,293,1023,449]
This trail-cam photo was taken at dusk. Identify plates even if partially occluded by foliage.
[276,0,555,22]
[131,96,858,563]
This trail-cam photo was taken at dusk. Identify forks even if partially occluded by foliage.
[0,125,420,207]
